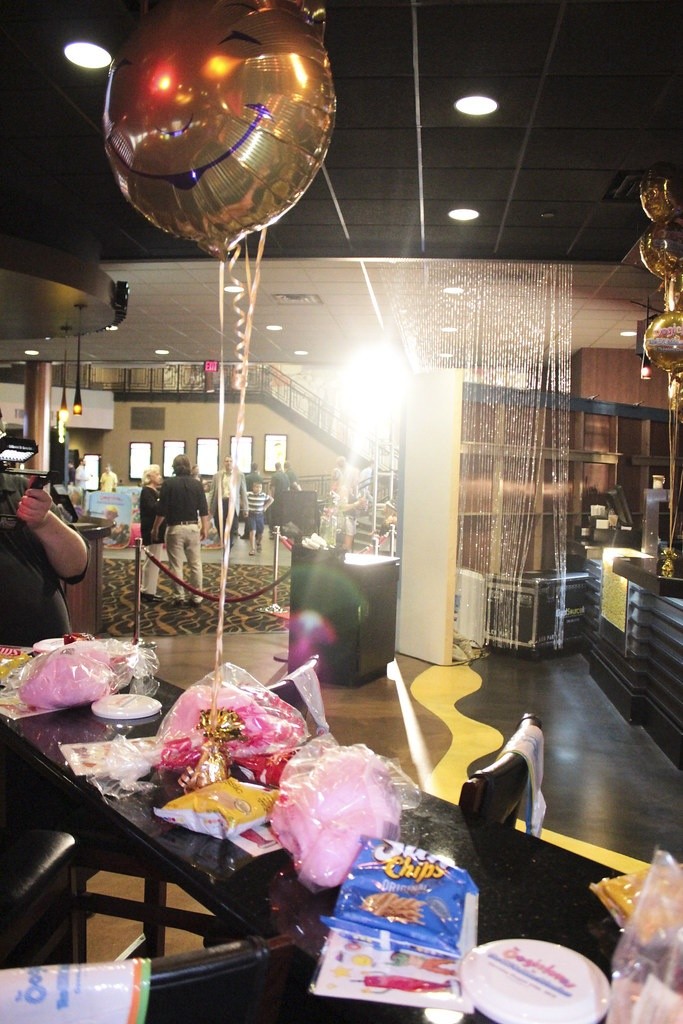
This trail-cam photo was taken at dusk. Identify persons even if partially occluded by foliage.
[100,464,117,492]
[139,456,209,605]
[69,457,88,492]
[332,456,399,551]
[209,456,274,554]
[0,473,91,645]
[270,462,301,533]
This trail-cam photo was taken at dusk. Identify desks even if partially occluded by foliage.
[60,523,111,636]
[0,676,626,1024]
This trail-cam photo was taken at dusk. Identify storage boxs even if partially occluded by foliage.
[484,569,597,660]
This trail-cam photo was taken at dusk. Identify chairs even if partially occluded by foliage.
[0,935,295,1023]
[0,831,87,970]
[265,655,321,719]
[459,713,542,828]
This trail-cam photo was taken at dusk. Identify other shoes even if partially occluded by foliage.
[174,599,185,608]
[234,531,240,536]
[189,599,202,607]
[257,542,261,553]
[240,534,248,538]
[142,591,163,603]
[249,550,255,555]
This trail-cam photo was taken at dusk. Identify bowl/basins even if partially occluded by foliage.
[588,516,607,528]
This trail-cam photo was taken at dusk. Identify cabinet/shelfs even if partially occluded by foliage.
[394,367,683,667]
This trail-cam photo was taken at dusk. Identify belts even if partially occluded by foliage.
[168,521,197,525]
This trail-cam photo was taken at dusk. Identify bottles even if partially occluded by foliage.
[609,508,615,515]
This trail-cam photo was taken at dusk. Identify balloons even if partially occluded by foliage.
[104,0,338,250]
[640,170,683,421]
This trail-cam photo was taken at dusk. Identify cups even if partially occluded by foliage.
[653,475,664,489]
[581,528,590,545]
[608,515,618,530]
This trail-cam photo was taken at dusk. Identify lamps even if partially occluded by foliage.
[0,437,60,489]
[59,326,71,421]
[73,304,85,415]
[641,296,653,380]
